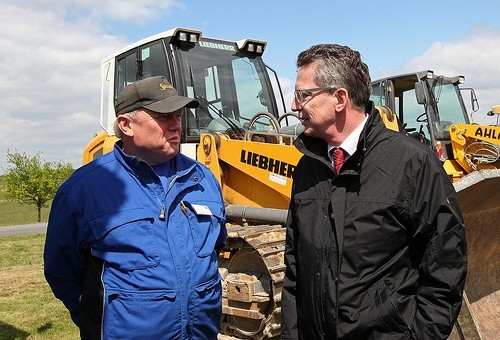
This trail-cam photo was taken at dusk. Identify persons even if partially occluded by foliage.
[42,74,232,339]
[279,41,469,339]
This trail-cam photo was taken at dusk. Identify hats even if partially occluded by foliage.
[115,75,200,116]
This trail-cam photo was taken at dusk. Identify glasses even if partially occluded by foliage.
[295,86,335,103]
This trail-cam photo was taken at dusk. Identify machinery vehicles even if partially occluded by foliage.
[83,27,499,340]
[370,70,499,183]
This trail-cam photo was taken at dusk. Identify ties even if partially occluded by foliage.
[331,148,346,174]
